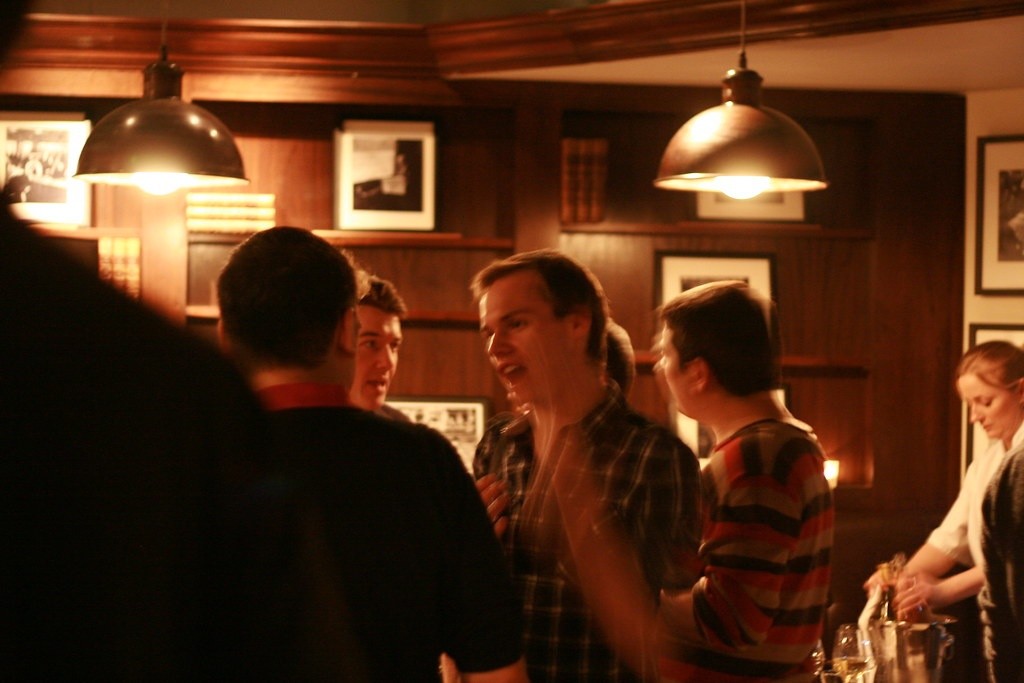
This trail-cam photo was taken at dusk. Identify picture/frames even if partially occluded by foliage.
[965,322,1024,476]
[382,395,491,478]
[695,190,809,220]
[653,249,782,352]
[335,121,436,232]
[0,113,93,230]
[974,134,1024,296]
[667,383,791,473]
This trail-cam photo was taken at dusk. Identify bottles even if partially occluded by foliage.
[878,551,929,683]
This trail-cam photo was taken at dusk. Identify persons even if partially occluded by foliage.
[0,0,363,683]
[470,250,701,683]
[863,341,1024,683]
[347,275,406,410]
[660,280,836,683]
[214,226,530,683]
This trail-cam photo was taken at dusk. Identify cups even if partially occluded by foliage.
[804,640,824,683]
[832,625,873,683]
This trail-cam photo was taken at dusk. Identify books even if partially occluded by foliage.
[185,192,276,233]
[98,238,140,298]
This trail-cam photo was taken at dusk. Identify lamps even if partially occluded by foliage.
[653,0,830,200]
[75,0,253,197]
